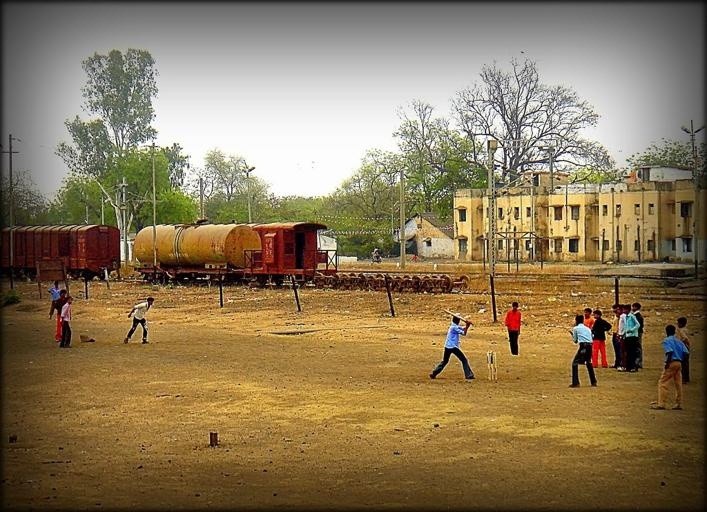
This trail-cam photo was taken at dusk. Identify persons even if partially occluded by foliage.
[430,316,476,379]
[124,297,154,343]
[651,325,689,409]
[372,246,380,260]
[48,281,73,348]
[676,317,691,383]
[582,302,644,371]
[569,315,597,387]
[504,302,521,354]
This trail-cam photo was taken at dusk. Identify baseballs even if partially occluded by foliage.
[456,313,461,316]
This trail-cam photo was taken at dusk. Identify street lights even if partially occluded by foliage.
[680,118,707,279]
[240,166,258,222]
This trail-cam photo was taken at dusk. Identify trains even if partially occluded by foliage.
[131,221,338,286]
[0,222,120,281]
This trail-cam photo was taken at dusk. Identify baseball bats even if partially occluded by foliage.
[444,309,468,323]
